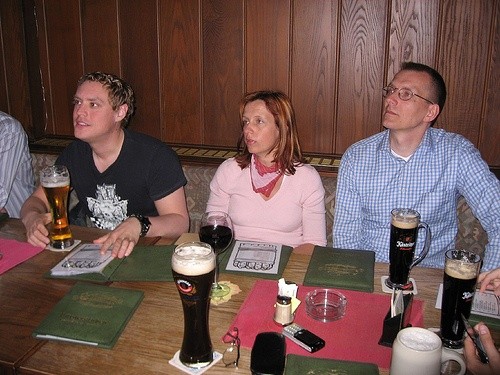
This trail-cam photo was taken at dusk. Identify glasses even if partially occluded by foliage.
[223,327,240,369]
[382,86,434,105]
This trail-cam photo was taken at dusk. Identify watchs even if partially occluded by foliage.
[131,213,151,236]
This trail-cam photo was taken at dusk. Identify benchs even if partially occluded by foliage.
[32,155,486,267]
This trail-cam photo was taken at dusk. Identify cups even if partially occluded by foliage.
[38,165,74,249]
[389,327,467,375]
[170,241,216,368]
[385,207,431,290]
[438,248,482,348]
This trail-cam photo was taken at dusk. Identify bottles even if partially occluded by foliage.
[274,295,293,326]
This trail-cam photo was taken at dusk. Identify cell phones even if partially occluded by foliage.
[460,313,488,364]
[282,323,325,352]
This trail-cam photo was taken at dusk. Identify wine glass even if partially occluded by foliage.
[198,211,233,297]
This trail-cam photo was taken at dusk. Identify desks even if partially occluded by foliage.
[0,217,161,372]
[16,238,500,375]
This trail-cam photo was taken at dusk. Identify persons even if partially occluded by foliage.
[0,111,35,219]
[463,268,500,375]
[332,61,500,273]
[205,90,327,256]
[20,71,190,258]
